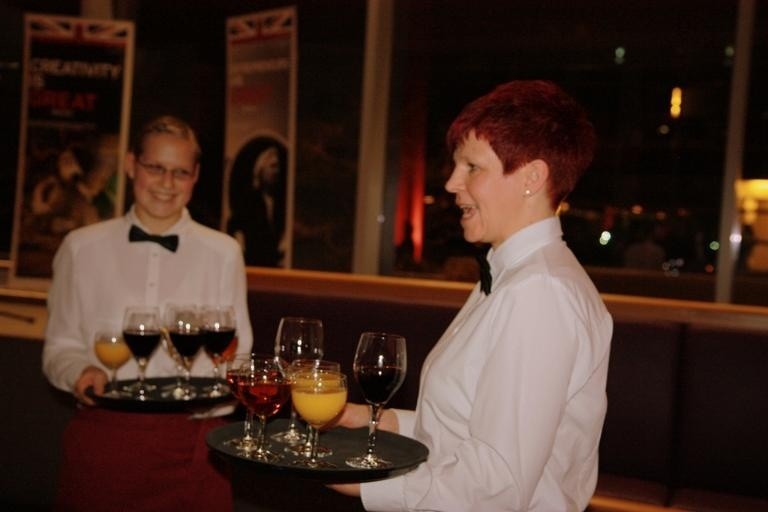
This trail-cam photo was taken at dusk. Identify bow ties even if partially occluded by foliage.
[478,258,492,297]
[128,224,180,253]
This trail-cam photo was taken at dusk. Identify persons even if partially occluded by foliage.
[313,78,617,511]
[37,110,256,510]
[226,148,285,265]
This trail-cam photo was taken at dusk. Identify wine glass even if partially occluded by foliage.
[93,330,130,398]
[123,300,240,400]
[225,313,408,469]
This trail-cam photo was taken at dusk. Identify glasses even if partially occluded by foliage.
[135,156,191,182]
